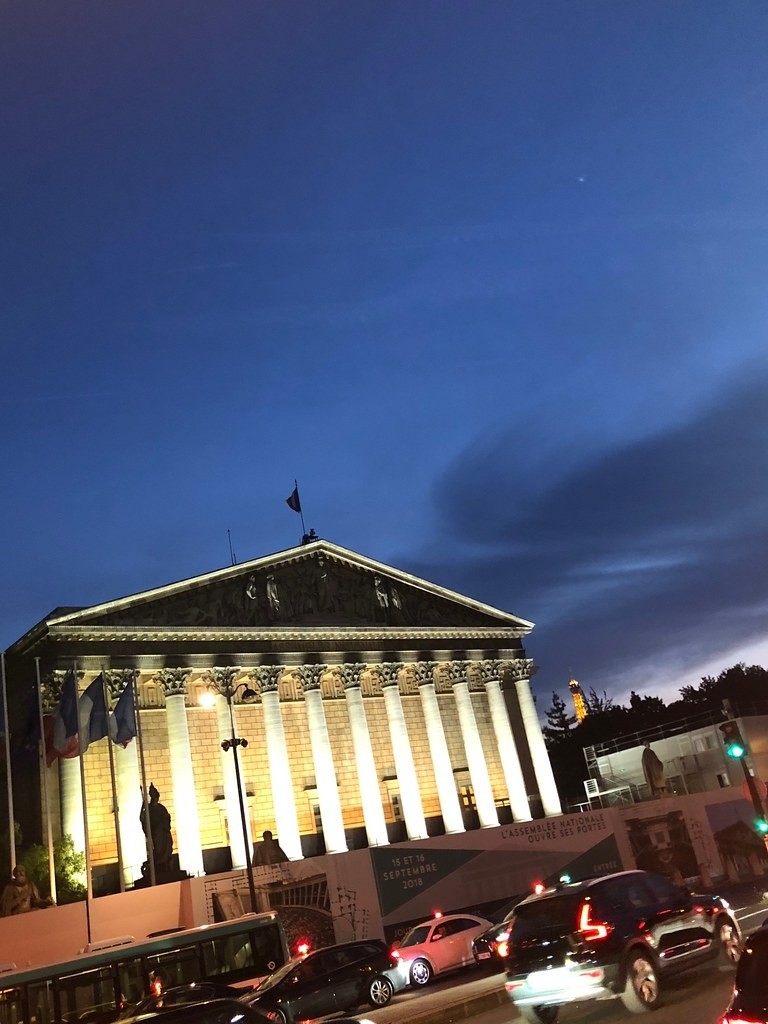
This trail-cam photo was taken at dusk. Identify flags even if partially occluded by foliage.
[42,670,80,768]
[78,672,107,753]
[110,674,137,748]
[285,487,300,513]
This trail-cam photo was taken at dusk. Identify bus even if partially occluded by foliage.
[0,911,293,1023]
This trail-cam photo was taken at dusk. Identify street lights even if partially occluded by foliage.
[211,669,263,910]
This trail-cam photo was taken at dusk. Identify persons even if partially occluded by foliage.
[250,831,290,868]
[0,864,56,916]
[140,781,175,884]
[642,739,665,796]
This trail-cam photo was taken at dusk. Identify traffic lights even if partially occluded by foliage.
[717,721,748,763]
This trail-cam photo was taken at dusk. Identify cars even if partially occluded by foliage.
[121,981,256,1018]
[386,913,494,991]
[113,997,275,1024]
[472,909,512,969]
[233,937,405,1024]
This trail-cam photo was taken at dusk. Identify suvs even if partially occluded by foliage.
[501,869,742,1024]
